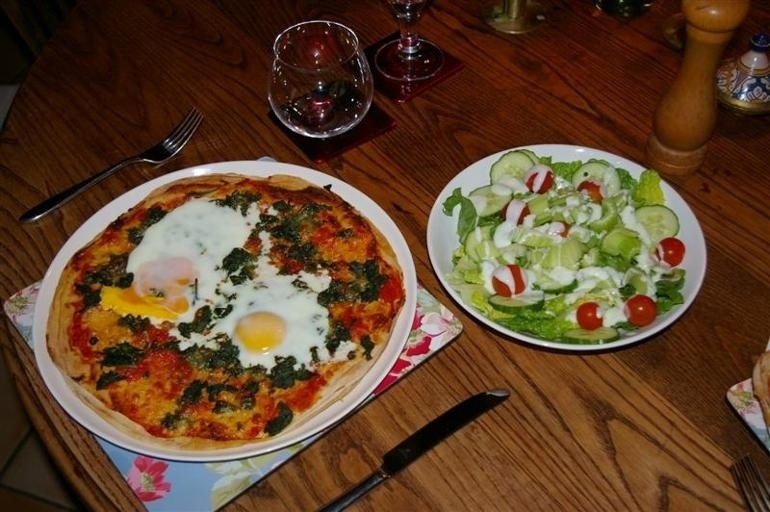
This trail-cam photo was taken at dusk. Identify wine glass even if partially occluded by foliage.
[378,1,449,87]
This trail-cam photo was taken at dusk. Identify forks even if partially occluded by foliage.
[724,451,769,510]
[20,105,211,230]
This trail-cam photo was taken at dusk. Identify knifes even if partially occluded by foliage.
[308,385,514,510]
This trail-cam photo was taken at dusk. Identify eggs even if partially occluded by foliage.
[101,196,260,337]
[217,262,349,372]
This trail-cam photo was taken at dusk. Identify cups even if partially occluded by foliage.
[268,19,375,146]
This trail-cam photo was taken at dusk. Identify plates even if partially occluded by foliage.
[423,142,708,352]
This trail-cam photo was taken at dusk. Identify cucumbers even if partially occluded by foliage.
[456,149,684,343]
